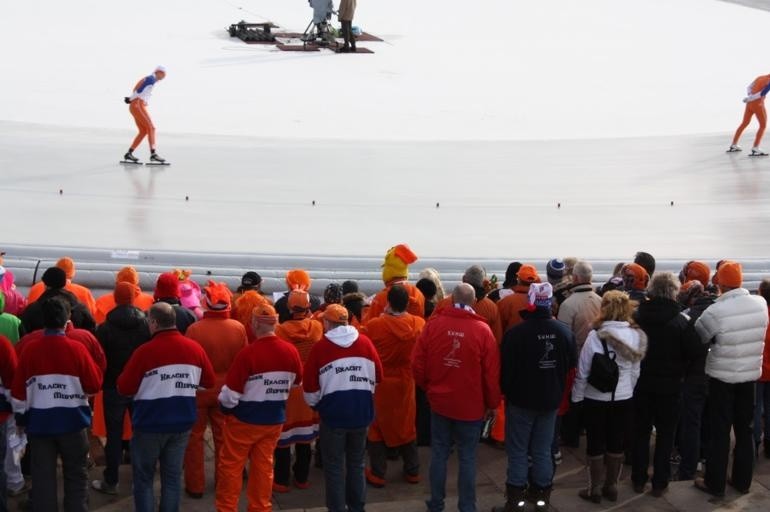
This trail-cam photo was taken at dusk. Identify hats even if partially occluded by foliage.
[621,263,649,289]
[685,261,708,287]
[718,263,741,288]
[42,258,75,288]
[154,270,358,321]
[505,258,578,305]
[115,266,138,304]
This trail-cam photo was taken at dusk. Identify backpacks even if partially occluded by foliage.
[588,328,619,392]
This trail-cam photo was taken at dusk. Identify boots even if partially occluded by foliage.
[579,453,626,502]
[92,480,118,494]
[493,484,552,512]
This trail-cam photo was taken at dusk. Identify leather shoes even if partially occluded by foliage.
[633,462,750,496]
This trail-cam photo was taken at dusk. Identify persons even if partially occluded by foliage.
[123,61,169,162]
[337,0,358,54]
[729,72,770,154]
[308,0,334,45]
[0,242,770,510]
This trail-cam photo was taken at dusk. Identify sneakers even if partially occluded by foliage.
[365,467,384,488]
[9,481,31,496]
